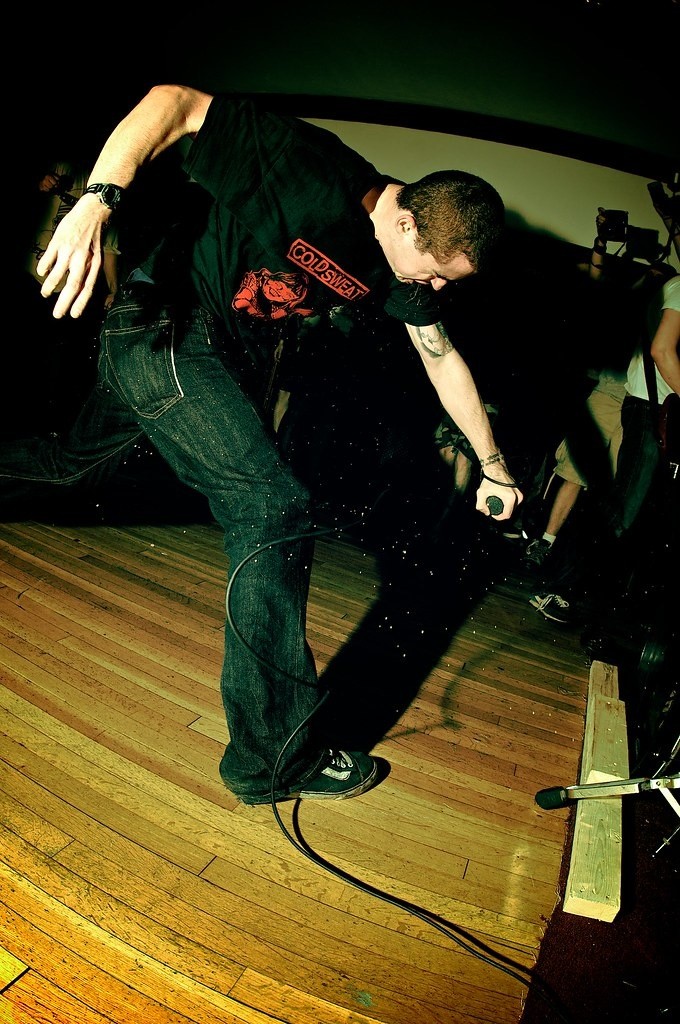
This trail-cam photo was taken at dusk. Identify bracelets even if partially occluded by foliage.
[484,474,517,488]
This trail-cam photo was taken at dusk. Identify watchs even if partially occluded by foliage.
[83,182,126,210]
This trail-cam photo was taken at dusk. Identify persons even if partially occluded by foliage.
[36,85,680,804]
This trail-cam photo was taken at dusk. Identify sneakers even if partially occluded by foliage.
[523,539,551,573]
[284,749,378,804]
[529,593,571,623]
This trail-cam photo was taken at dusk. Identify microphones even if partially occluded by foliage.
[484,495,503,516]
